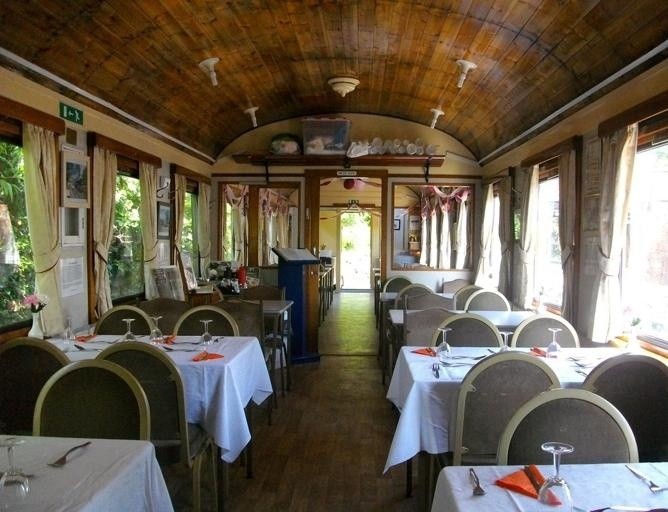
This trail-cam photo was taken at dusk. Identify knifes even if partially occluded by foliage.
[523,464,546,500]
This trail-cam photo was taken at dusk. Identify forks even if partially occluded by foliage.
[624,463,666,495]
[470,467,487,497]
[47,438,91,470]
[432,365,440,378]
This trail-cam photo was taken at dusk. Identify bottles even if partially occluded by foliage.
[237,264,248,284]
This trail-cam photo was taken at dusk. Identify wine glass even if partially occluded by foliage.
[197,318,216,347]
[61,317,78,347]
[498,331,514,353]
[434,326,453,364]
[544,326,564,366]
[535,440,573,507]
[122,318,135,341]
[0,437,31,512]
[148,316,164,346]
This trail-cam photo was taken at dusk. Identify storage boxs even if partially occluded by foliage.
[299,119,351,157]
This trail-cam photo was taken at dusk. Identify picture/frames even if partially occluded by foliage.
[155,201,173,240]
[60,151,93,209]
[393,219,400,231]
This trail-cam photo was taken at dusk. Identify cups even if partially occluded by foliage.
[368,137,438,155]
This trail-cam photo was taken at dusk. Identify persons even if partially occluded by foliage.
[158,209,167,233]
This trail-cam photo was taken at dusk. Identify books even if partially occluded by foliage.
[272,247,319,261]
[196,283,213,294]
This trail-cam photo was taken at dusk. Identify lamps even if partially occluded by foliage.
[328,77,360,98]
[243,105,259,128]
[427,107,445,130]
[455,59,477,89]
[198,57,219,86]
